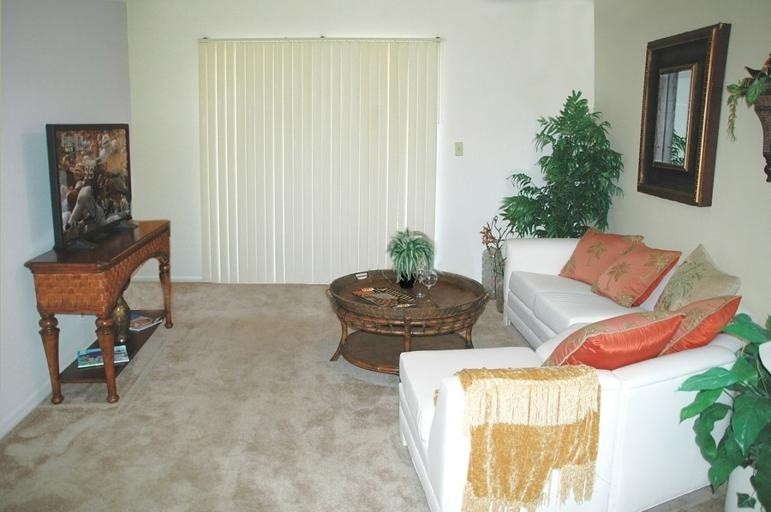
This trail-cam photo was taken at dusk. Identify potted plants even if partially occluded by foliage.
[388,226,435,288]
[677,312,771,512]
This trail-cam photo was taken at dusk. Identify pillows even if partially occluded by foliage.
[540,227,744,371]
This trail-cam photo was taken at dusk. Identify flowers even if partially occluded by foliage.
[726,52,771,140]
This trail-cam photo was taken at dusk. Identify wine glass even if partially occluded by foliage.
[411,266,427,299]
[421,271,438,301]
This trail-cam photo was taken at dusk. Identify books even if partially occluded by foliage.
[127,311,163,331]
[77,345,130,368]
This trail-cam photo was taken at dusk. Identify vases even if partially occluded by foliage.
[755,95,771,183]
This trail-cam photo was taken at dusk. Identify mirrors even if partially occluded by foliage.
[636,21,734,210]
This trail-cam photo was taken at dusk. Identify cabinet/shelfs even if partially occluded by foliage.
[24,218,173,403]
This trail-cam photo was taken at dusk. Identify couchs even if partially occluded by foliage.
[398,238,751,511]
